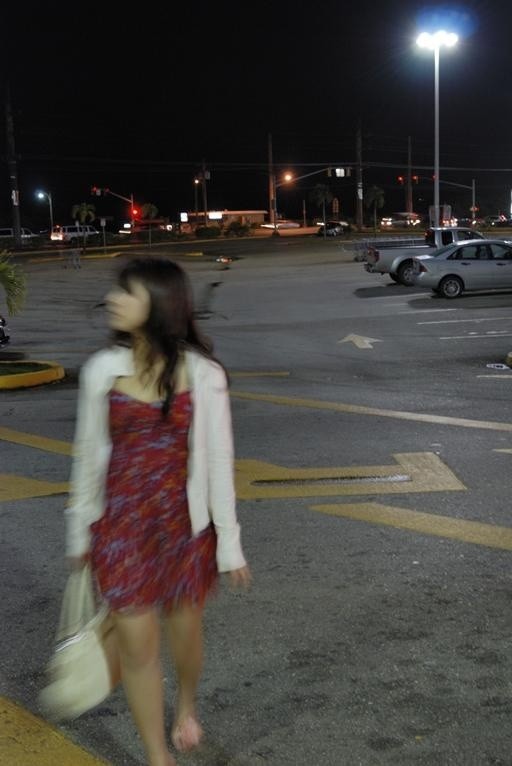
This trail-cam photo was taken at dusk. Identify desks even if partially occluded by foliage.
[195,180,207,225]
[38,190,54,227]
[416,31,459,228]
[274,175,291,231]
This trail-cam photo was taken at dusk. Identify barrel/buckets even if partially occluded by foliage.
[172,711,204,753]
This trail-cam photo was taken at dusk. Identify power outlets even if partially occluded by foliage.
[365,228,485,286]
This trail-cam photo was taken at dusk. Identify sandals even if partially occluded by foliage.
[50,225,100,242]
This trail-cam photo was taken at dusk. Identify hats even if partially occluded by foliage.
[318,222,344,237]
[409,239,512,299]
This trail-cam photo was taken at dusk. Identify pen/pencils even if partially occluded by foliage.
[1,227,40,237]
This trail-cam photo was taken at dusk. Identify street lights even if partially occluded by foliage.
[43,603,122,719]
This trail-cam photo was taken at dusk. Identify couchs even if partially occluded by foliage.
[413,176,418,184]
[399,176,404,185]
[133,210,138,216]
[91,185,109,196]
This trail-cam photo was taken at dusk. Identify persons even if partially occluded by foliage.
[65,250,256,766]
